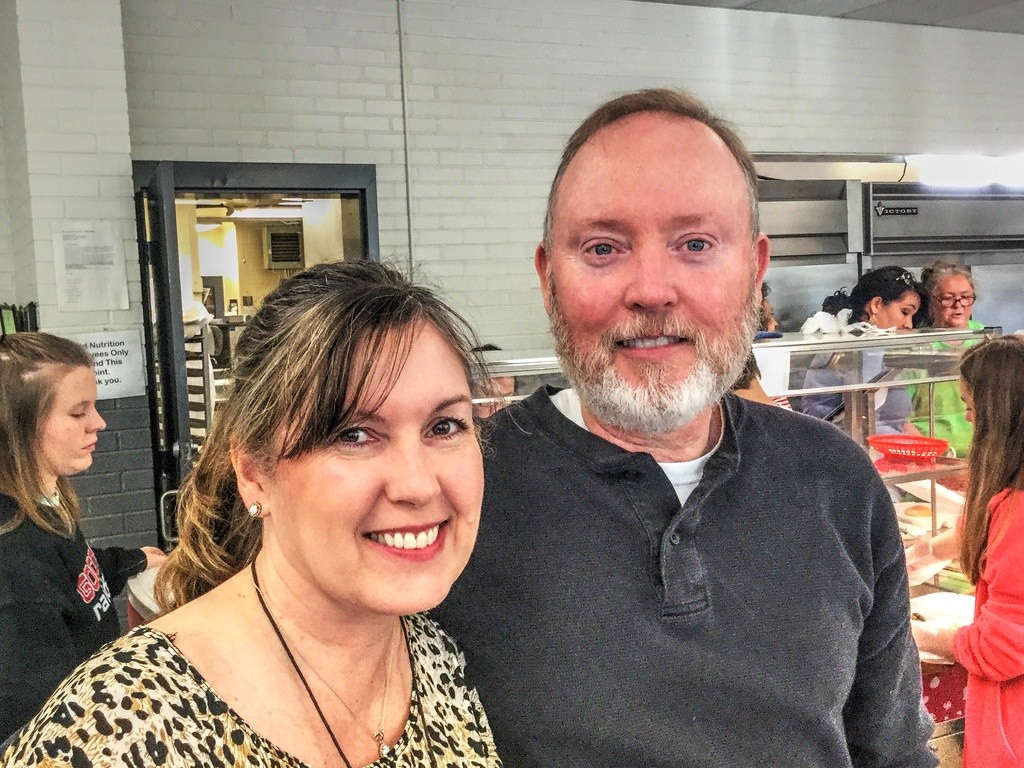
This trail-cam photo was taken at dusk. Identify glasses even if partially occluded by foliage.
[932,292,976,308]
[895,271,917,286]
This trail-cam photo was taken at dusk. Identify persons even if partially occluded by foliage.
[731,282,792,410]
[469,344,518,418]
[431,89,940,768]
[0,332,168,746]
[905,336,1024,768]
[1,259,506,768]
[901,263,989,458]
[802,264,922,502]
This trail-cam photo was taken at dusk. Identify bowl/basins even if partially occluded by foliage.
[893,501,947,532]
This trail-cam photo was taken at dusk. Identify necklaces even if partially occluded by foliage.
[252,550,438,768]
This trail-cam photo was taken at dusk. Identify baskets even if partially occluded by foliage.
[867,434,949,462]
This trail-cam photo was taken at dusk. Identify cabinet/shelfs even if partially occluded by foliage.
[470,326,1003,596]
